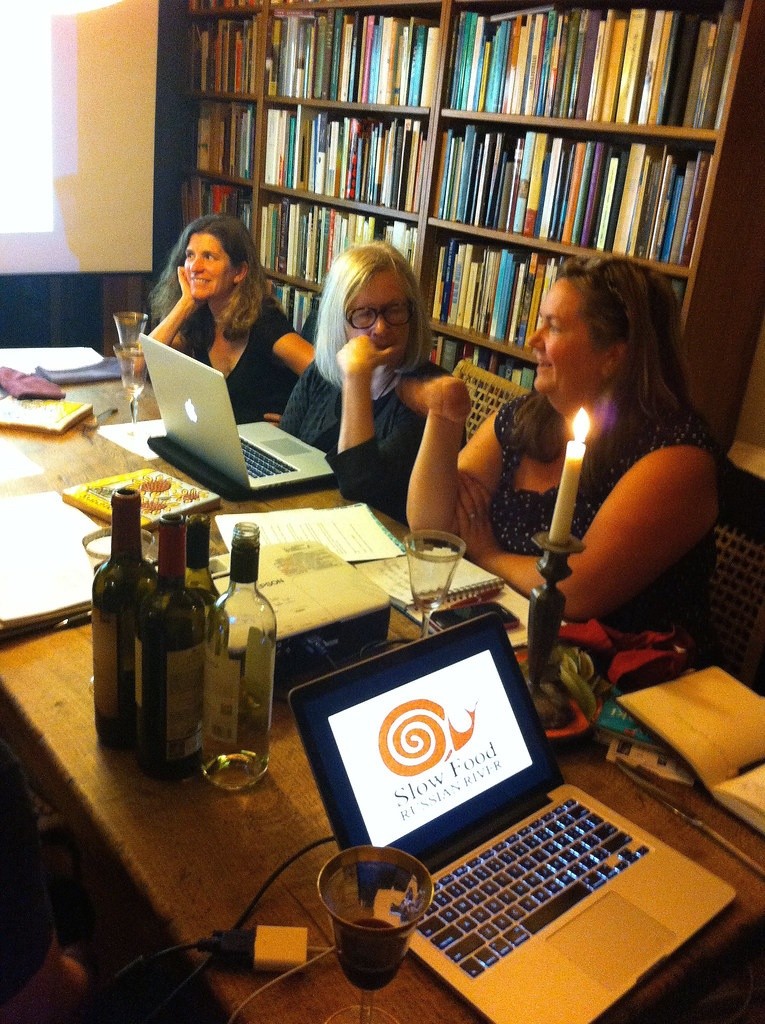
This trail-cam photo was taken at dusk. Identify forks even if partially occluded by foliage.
[84,408,118,431]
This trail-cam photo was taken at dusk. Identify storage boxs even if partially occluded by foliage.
[206,537,391,703]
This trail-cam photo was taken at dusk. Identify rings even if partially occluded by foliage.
[469,512,477,521]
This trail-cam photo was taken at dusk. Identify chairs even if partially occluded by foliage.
[450,358,531,444]
[707,516,765,688]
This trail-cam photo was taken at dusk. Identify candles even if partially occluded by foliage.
[548,407,590,545]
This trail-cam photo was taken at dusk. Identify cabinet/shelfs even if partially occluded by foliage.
[187,0,765,458]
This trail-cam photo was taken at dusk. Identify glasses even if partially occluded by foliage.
[344,304,415,329]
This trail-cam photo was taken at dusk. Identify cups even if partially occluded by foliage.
[114,311,149,350]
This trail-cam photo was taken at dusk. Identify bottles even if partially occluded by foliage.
[201,522,277,790]
[133,514,229,783]
[91,488,159,748]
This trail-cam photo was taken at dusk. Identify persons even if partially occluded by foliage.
[278,242,466,526]
[134,213,297,424]
[404,253,726,672]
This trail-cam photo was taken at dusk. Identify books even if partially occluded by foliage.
[197,102,256,180]
[264,0,440,107]
[353,548,567,648]
[432,238,563,347]
[266,279,322,333]
[181,178,252,232]
[429,336,535,391]
[265,103,427,213]
[446,0,741,130]
[432,124,710,266]
[613,666,765,836]
[260,197,418,284]
[188,0,262,93]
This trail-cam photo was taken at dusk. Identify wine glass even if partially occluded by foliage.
[316,845,433,1024]
[113,343,147,422]
[405,530,466,637]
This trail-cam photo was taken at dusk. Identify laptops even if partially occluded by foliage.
[138,332,335,490]
[286,611,736,1024]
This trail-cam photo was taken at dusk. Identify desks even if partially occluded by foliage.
[0,346,764,1024]
